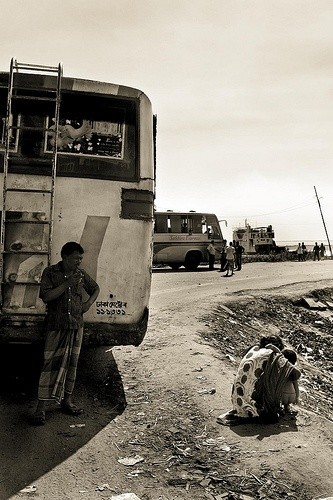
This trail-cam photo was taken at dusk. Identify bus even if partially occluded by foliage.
[152,210,227,271]
[0,58,157,348]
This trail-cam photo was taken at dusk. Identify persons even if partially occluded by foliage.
[220,240,245,275]
[34,242,100,425]
[207,240,219,271]
[297,242,325,262]
[204,228,212,235]
[232,335,301,418]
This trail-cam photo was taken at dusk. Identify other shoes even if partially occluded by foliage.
[60,403,82,416]
[34,410,45,424]
[226,273,235,276]
